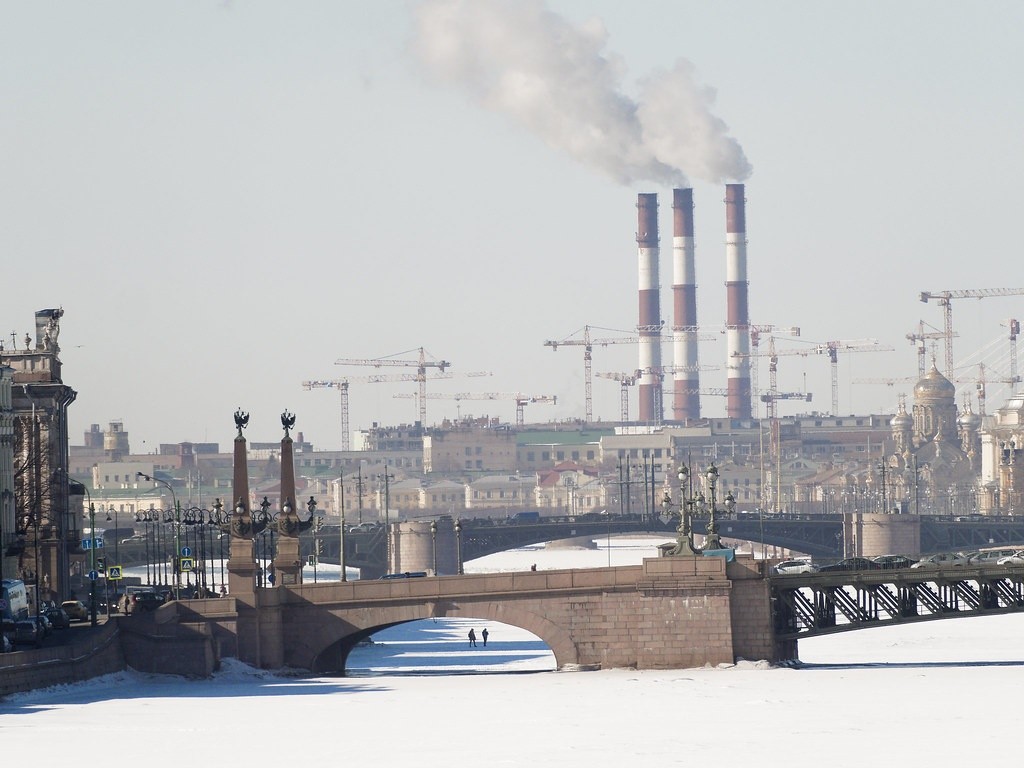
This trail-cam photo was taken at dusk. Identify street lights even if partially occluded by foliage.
[137,471,181,600]
[106,509,118,601]
[51,472,95,652]
[453,518,463,574]
[662,462,702,556]
[430,520,437,577]
[695,462,737,550]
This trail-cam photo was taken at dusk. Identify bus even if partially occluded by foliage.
[318,524,348,533]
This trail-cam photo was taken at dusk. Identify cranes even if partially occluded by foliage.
[302,347,558,451]
[904,287,1023,415]
[546,324,895,435]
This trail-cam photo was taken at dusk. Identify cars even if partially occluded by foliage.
[350,522,378,533]
[0,600,88,653]
[575,512,602,522]
[119,530,229,543]
[770,549,1024,576]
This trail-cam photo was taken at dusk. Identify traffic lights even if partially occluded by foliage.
[307,555,314,566]
[315,517,324,532]
[317,539,324,555]
[98,557,104,573]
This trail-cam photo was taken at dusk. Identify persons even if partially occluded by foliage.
[167,591,173,602]
[469,629,477,647]
[482,628,489,646]
[532,564,537,571]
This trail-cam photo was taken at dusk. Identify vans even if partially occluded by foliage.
[118,592,168,615]
[100,527,134,544]
[511,511,540,518]
